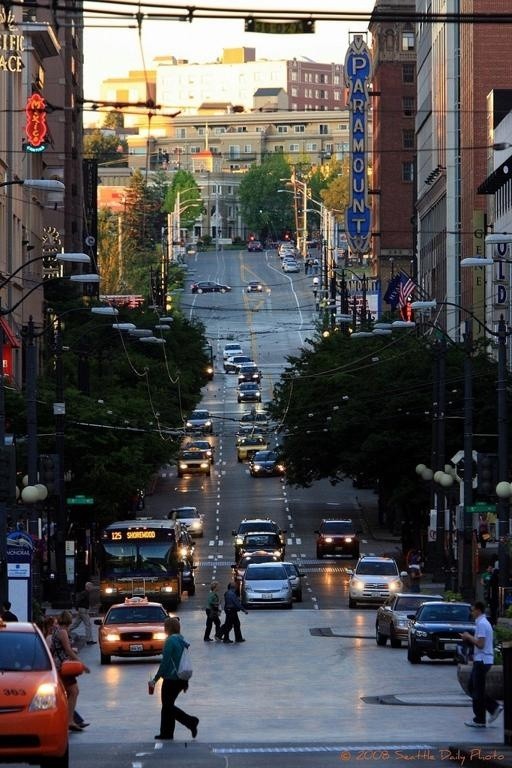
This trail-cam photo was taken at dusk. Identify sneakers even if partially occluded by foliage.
[191,717,199,738]
[69,725,82,731]
[488,705,504,723]
[464,720,486,727]
[204,633,245,643]
[80,724,89,728]
[155,735,173,739]
[86,641,96,645]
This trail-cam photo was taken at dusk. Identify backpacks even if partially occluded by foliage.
[170,635,192,681]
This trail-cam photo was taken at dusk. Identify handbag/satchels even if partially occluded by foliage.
[209,607,221,618]
[455,644,467,665]
[60,661,83,678]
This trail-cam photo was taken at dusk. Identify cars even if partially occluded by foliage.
[189,280,232,295]
[245,280,265,290]
[246,241,264,253]
[372,589,446,647]
[177,408,287,480]
[406,600,481,664]
[1,616,87,768]
[275,238,301,275]
[221,341,264,404]
[93,596,182,663]
[163,501,406,612]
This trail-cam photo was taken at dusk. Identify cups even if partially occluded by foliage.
[148,681,155,694]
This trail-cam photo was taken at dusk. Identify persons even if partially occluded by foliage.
[458,599,503,727]
[0,582,96,731]
[204,582,248,643]
[481,566,493,598]
[148,617,199,740]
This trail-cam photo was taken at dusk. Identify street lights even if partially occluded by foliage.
[279,176,308,260]
[161,182,212,267]
[276,190,302,198]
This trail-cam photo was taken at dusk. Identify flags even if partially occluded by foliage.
[384,272,415,312]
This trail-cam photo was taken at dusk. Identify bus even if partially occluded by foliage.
[196,333,217,383]
[97,512,184,610]
